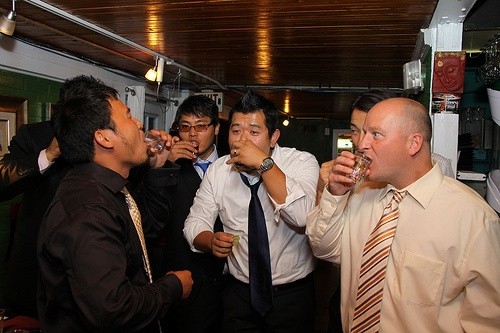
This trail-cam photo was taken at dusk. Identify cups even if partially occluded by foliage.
[343,151,372,188]
[234,154,244,166]
[187,139,199,157]
[7,329,29,333]
[227,234,240,248]
[143,129,165,152]
[0,309,5,333]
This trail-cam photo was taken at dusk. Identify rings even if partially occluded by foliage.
[234,148,237,155]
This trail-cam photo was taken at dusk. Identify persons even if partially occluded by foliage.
[148,96,233,333]
[316,90,455,205]
[33,85,195,333]
[183,89,320,333]
[0,76,114,318]
[306,97,500,333]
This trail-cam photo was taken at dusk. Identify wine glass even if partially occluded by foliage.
[478,32,500,85]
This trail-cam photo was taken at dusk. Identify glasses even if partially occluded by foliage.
[177,122,213,132]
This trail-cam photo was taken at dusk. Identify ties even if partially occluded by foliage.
[194,161,212,174]
[120,186,162,333]
[237,171,274,317]
[349,189,409,333]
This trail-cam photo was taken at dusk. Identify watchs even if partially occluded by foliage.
[257,157,274,174]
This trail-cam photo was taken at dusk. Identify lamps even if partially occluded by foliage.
[145,57,160,81]
[283,115,291,126]
[402,59,426,94]
[0,0,19,36]
